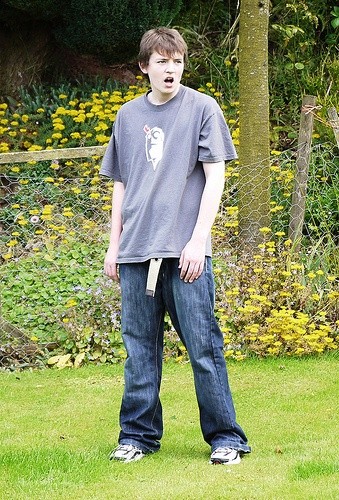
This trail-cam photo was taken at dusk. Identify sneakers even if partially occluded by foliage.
[109,443,144,463]
[210,443,243,465]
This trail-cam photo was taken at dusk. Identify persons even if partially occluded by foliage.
[100,27,251,463]
